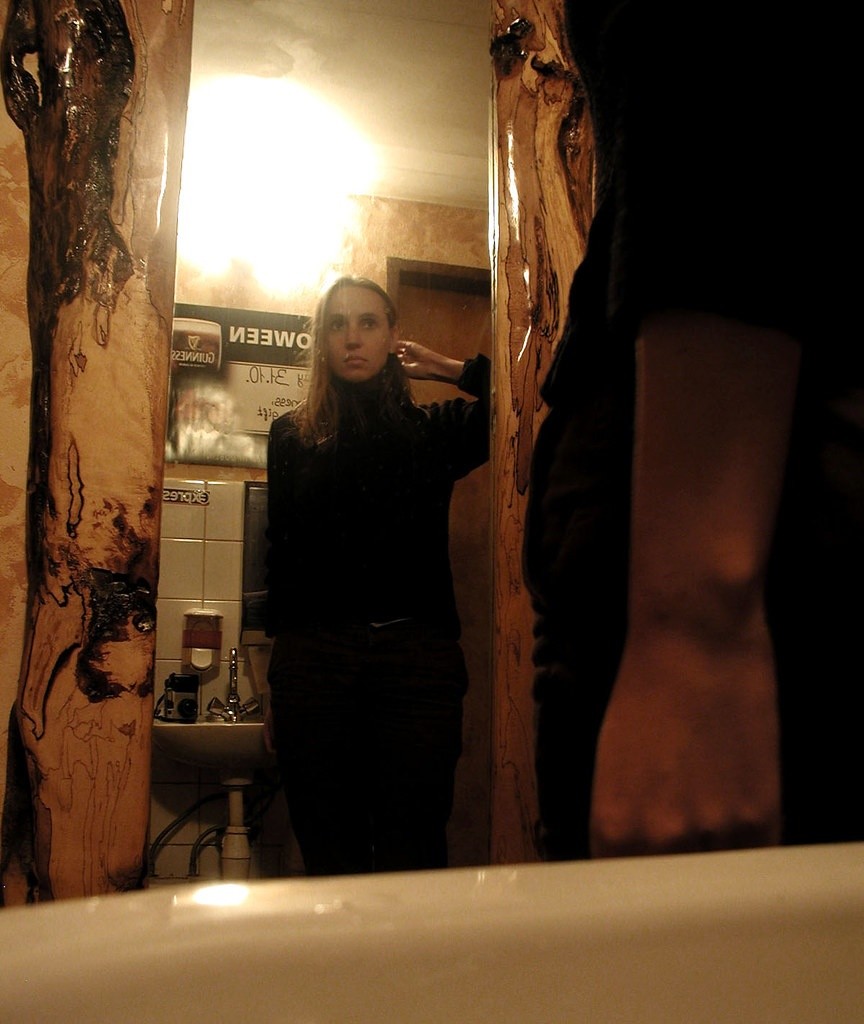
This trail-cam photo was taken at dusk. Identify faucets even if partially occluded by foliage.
[206,647,259,724]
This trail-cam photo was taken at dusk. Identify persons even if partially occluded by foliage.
[520,0,864,863]
[260,275,492,876]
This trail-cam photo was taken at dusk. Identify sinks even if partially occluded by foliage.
[151,709,265,768]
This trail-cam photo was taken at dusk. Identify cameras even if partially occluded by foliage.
[161,673,200,723]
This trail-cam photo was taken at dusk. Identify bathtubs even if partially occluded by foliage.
[0,842,863,1023]
